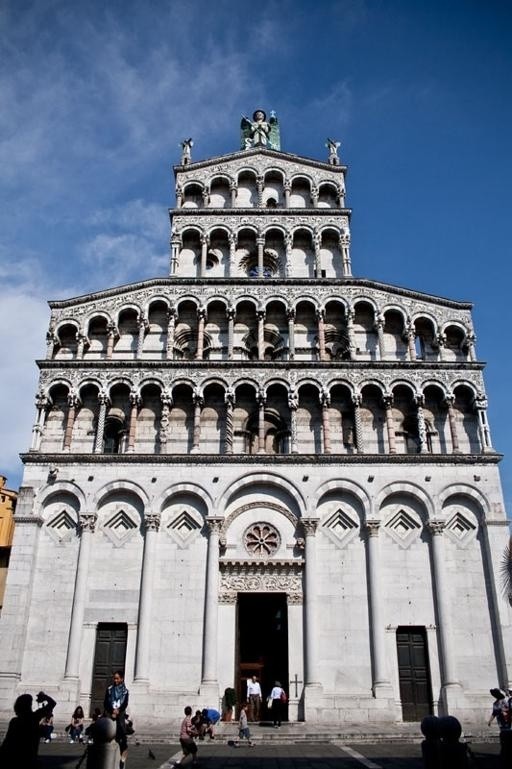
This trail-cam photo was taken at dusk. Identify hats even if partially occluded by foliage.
[490,688,505,698]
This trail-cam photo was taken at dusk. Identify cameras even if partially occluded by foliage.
[36,695,44,703]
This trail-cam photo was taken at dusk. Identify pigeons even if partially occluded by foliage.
[135,738,141,747]
[148,748,156,760]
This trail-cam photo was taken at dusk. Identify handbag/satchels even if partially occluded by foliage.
[281,692,286,703]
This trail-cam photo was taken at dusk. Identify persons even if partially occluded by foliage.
[440,715,474,769]
[420,715,443,769]
[0,691,135,769]
[486,685,512,764]
[171,705,199,769]
[178,674,287,747]
[104,669,128,749]
[250,110,270,147]
[326,139,341,165]
[180,139,193,165]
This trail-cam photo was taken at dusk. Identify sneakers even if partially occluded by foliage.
[44,739,49,743]
[69,740,74,743]
